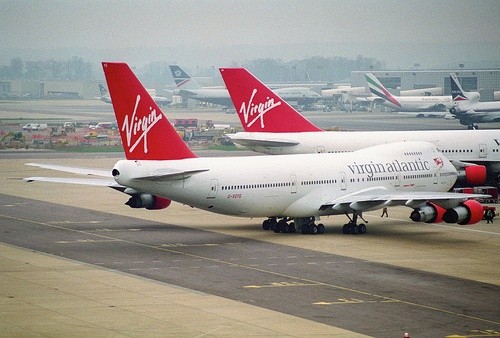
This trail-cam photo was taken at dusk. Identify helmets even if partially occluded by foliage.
[404,333,408,337]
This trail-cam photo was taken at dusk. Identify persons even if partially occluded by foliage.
[485,207,493,224]
[381,207,389,217]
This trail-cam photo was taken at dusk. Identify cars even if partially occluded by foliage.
[84,132,108,139]
[22,123,48,131]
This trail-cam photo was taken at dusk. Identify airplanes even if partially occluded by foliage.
[417,73,500,130]
[364,73,500,117]
[93,83,173,107]
[170,65,323,109]
[5,62,492,235]
[217,67,500,186]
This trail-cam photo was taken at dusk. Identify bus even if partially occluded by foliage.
[453,187,498,203]
